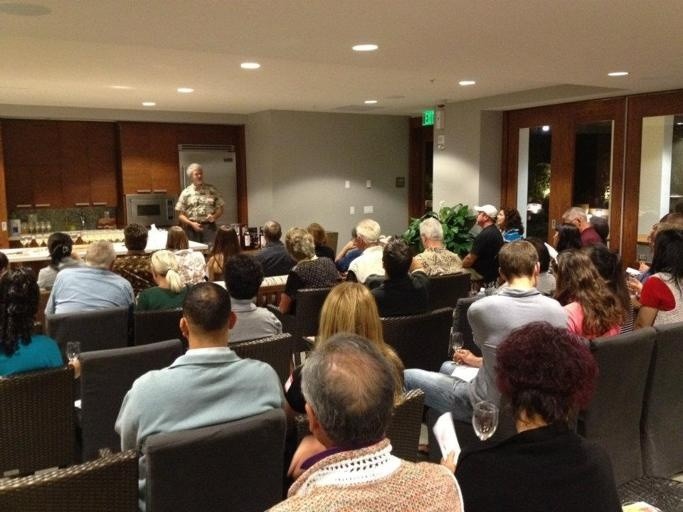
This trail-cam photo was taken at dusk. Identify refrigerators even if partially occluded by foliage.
[177,143,238,236]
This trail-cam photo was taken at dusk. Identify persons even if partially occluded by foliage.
[1,162,683,512]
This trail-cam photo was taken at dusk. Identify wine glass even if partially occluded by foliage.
[66,342,80,361]
[639,255,647,263]
[20,236,49,248]
[471,400,499,443]
[18,220,52,235]
[450,332,464,366]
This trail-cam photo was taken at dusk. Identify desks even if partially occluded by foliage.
[1,234,210,275]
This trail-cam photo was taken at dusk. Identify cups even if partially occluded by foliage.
[625,276,638,300]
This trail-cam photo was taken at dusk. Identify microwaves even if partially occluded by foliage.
[125,193,180,228]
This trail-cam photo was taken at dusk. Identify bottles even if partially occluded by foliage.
[477,286,487,298]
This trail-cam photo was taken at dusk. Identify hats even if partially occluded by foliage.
[473,204,498,222]
